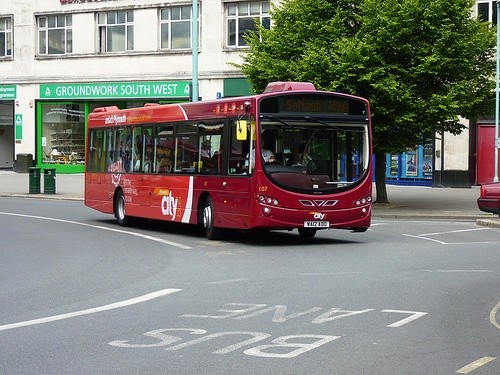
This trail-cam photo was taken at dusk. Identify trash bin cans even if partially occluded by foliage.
[29,167,41,194]
[43,168,56,194]
[16,153,33,173]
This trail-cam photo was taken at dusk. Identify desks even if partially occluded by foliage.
[42,161,85,165]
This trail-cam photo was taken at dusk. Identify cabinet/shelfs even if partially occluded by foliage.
[50,132,85,160]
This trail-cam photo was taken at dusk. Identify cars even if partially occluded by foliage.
[476,181,500,218]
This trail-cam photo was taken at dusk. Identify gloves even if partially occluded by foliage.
[268,158,275,162]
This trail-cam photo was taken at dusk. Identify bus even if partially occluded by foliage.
[84,81,373,241]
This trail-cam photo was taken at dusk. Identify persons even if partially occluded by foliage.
[287,143,300,166]
[391,149,433,179]
[292,142,316,175]
[244,138,275,174]
[49,139,75,163]
[91,129,243,175]
[351,150,358,180]
